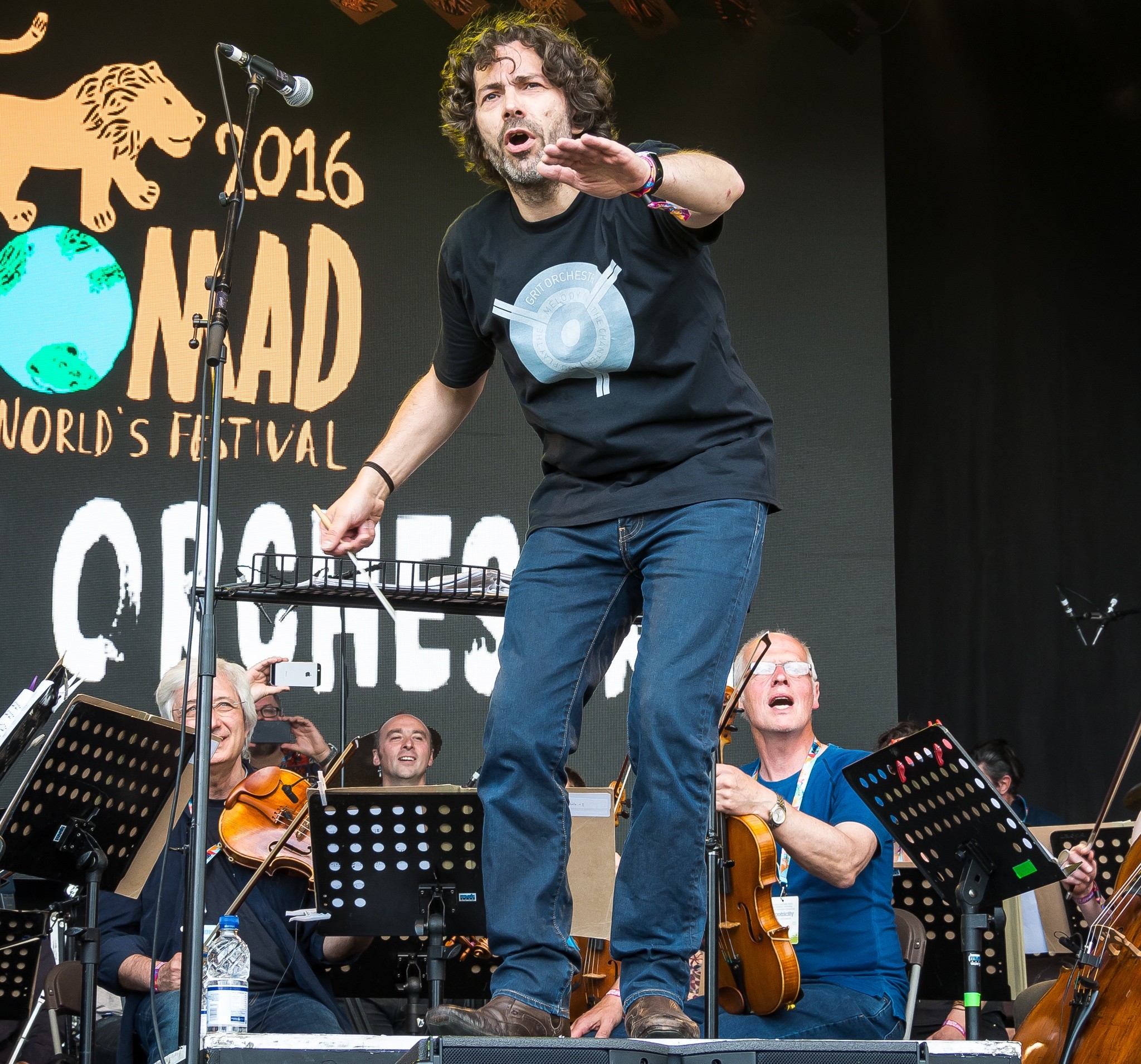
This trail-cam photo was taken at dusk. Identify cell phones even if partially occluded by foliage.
[270,661,322,687]
[250,720,291,744]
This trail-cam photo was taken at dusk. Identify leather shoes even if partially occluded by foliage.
[623,994,700,1039]
[426,996,572,1038]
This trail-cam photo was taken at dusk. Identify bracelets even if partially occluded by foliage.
[319,751,336,768]
[362,460,395,495]
[154,961,168,993]
[1073,889,1095,905]
[629,155,691,220]
[607,988,620,997]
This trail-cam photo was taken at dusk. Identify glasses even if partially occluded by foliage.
[173,699,247,721]
[256,707,281,718]
[749,661,812,676]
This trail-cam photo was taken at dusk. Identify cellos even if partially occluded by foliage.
[1012,833,1141,1063]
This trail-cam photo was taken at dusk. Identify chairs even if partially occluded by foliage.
[889,909,927,1041]
[44,960,85,1064]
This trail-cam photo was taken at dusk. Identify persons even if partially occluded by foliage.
[321,13,780,1028]
[77,631,1141,1064]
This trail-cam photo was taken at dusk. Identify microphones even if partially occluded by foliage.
[1092,595,1120,645]
[216,42,313,108]
[1057,593,1088,647]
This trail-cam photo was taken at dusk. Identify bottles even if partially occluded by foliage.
[206,915,251,1034]
[200,945,210,1036]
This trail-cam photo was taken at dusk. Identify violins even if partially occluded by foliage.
[218,764,493,963]
[705,685,802,1018]
[568,780,622,1026]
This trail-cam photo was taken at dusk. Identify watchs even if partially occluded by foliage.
[767,790,786,831]
[634,150,666,195]
